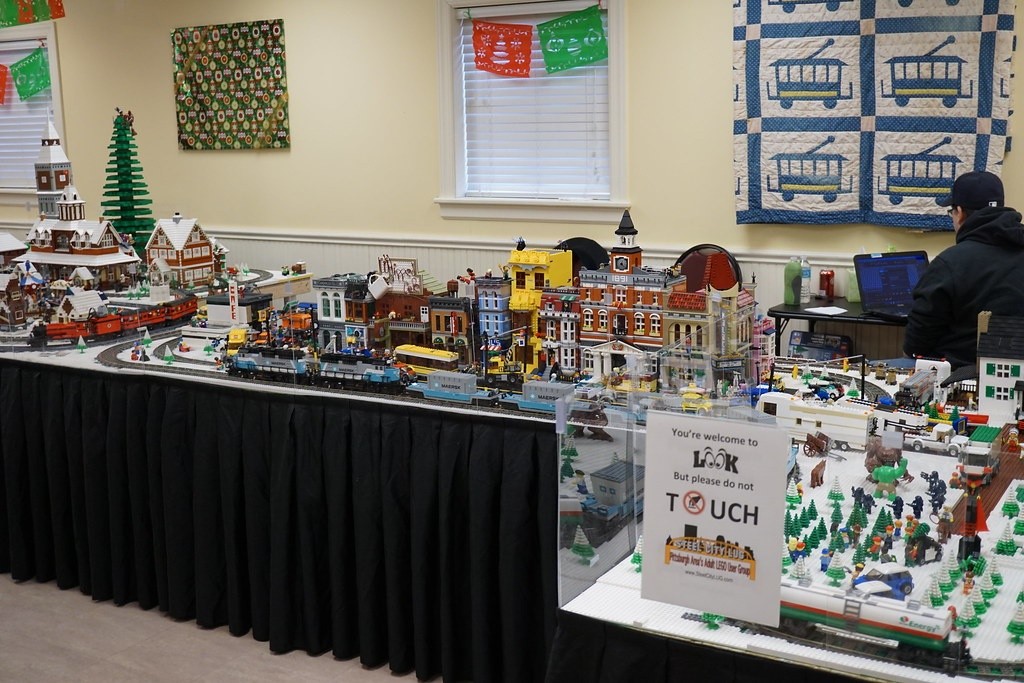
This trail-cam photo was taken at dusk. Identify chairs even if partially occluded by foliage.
[674,245,743,290]
[554,238,610,277]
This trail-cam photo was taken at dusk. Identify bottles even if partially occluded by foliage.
[784,255,813,307]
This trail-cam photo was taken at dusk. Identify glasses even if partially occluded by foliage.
[947,208,957,217]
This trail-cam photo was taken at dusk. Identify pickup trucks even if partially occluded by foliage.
[902,424,968,459]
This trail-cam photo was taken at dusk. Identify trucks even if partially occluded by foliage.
[892,370,939,409]
[225,329,248,358]
[957,427,1004,487]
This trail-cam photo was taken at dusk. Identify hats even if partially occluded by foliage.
[935,170,1004,210]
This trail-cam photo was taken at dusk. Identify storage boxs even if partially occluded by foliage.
[787,330,854,362]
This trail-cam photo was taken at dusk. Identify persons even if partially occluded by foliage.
[853,471,947,519]
[901,172,1024,368]
[1007,427,1019,451]
[790,515,918,577]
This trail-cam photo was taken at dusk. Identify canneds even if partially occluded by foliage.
[819,270,834,298]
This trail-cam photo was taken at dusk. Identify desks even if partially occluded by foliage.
[0,270,558,683]
[767,296,908,356]
[195,270,313,308]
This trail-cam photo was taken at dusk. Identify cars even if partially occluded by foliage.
[254,332,271,347]
[681,393,712,417]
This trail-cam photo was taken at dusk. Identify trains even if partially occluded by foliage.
[227,344,655,427]
[25,289,198,348]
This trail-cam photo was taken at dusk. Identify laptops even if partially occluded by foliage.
[854,250,930,323]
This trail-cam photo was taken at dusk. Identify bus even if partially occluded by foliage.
[392,345,461,381]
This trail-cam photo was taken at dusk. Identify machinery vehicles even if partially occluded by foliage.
[482,343,524,385]
